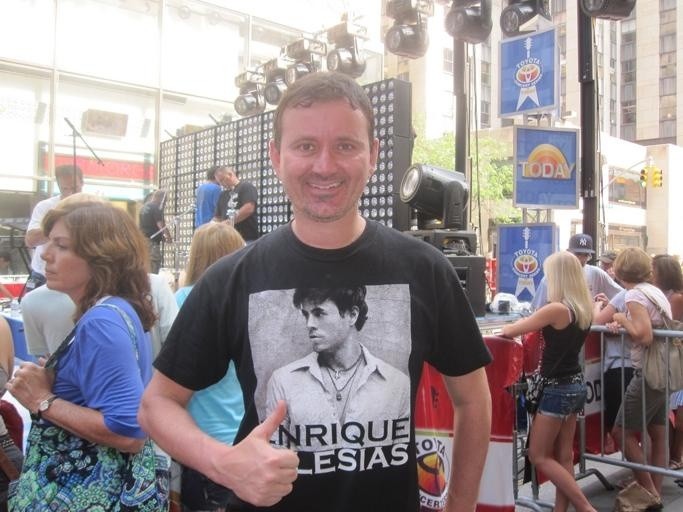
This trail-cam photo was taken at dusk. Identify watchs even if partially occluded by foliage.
[36,393,61,417]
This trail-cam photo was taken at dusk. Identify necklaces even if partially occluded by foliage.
[323,347,364,400]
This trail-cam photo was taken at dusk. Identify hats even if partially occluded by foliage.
[41,192,105,238]
[593,250,620,267]
[568,234,597,255]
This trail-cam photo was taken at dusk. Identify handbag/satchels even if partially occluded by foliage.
[518,349,570,415]
[8,304,171,508]
[626,286,683,392]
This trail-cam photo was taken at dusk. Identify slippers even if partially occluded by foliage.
[669,459,683,472]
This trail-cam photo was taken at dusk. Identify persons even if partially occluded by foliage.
[597,248,622,283]
[613,248,672,507]
[137,188,173,260]
[20,163,84,297]
[647,252,683,470]
[7,202,170,512]
[213,166,258,244]
[266,285,411,473]
[502,250,597,512]
[529,233,624,320]
[193,163,221,229]
[139,192,153,206]
[168,221,248,446]
[593,285,633,432]
